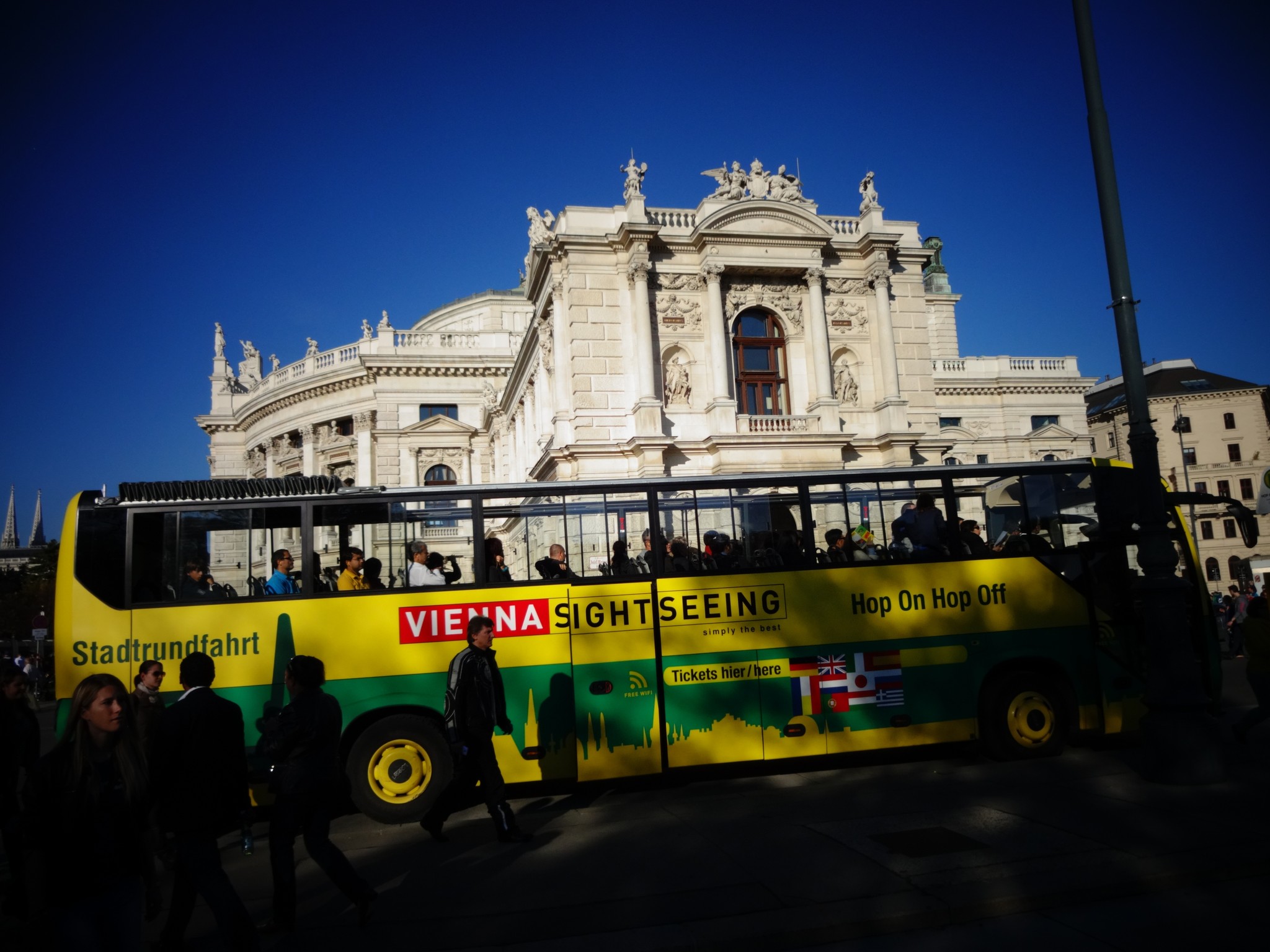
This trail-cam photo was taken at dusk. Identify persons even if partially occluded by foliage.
[1241,595,1270,727]
[765,164,815,205]
[675,365,690,388]
[253,447,260,457]
[706,160,748,200]
[1,642,378,951]
[376,309,390,328]
[214,321,226,358]
[361,318,373,338]
[172,488,1053,598]
[330,420,338,431]
[666,355,688,403]
[238,337,258,375]
[268,353,281,372]
[304,336,319,355]
[282,434,292,445]
[619,157,649,196]
[1209,568,1269,655]
[418,616,535,845]
[834,357,853,403]
[859,171,880,207]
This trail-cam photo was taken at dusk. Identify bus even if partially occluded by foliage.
[55,467,1261,824]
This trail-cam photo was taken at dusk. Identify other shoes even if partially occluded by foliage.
[497,829,534,845]
[419,816,448,844]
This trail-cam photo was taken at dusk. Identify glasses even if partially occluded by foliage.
[490,548,503,554]
[349,556,366,561]
[143,670,166,678]
[1014,528,1022,535]
[279,556,295,561]
[1037,523,1041,526]
[837,537,846,541]
[973,526,980,530]
[661,540,669,545]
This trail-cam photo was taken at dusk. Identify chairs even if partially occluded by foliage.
[157,530,1018,604]
